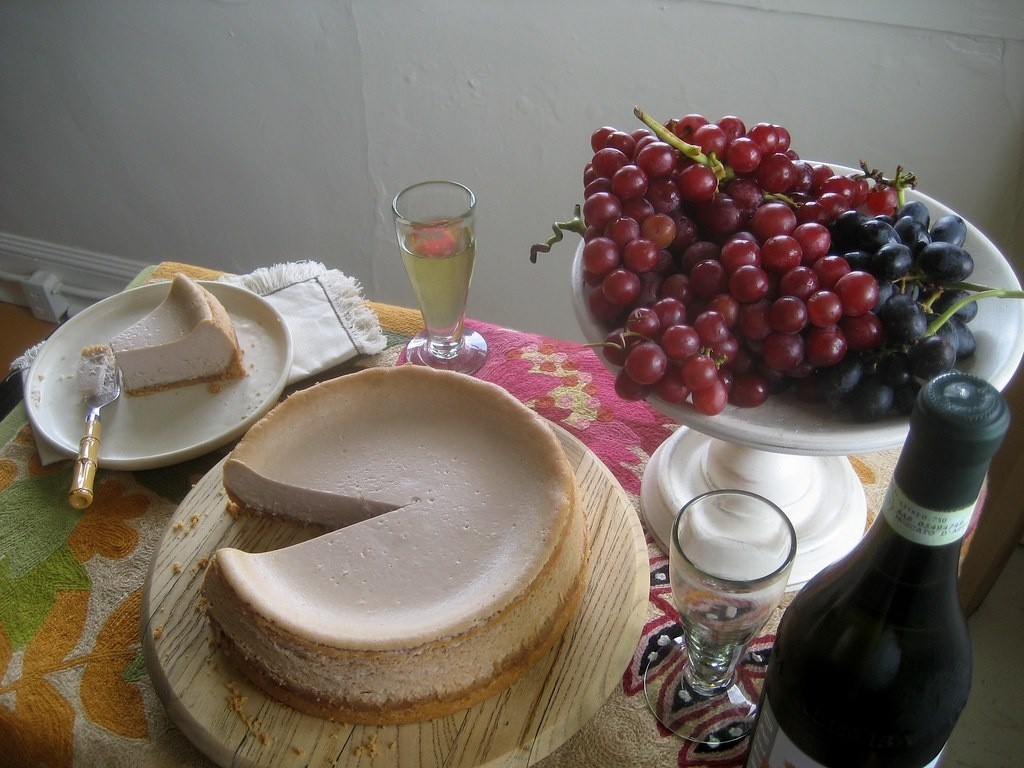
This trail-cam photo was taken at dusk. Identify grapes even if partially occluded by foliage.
[583,106,977,420]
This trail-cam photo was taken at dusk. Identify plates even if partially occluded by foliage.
[23,280,293,471]
[141,413,649,767]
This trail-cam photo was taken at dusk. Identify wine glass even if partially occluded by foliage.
[392,179,490,375]
[644,487,798,752]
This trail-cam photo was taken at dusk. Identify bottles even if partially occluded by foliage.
[741,373,1011,768]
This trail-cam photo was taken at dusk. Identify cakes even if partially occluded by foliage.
[109,276,249,394]
[201,364,591,727]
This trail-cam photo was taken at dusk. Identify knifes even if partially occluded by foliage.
[68,345,119,509]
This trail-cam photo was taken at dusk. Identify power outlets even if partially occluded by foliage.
[19,270,69,324]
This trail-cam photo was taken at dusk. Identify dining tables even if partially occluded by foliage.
[0,263,986,768]
